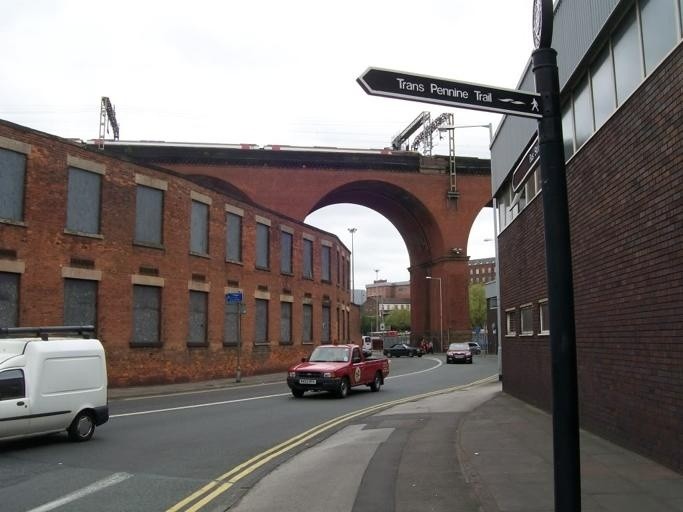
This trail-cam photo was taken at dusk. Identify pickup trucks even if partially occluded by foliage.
[287,343,390,396]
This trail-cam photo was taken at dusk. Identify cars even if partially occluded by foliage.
[416,337,434,353]
[446,343,473,363]
[383,343,422,358]
[464,342,481,355]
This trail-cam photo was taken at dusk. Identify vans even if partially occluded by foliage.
[0,336,109,443]
[362,335,372,350]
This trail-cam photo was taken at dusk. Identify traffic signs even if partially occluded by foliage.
[225,293,243,303]
[355,68,543,119]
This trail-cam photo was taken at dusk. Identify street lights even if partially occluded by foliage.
[348,227,356,304]
[426,277,444,352]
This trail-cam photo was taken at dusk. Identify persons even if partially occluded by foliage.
[419,336,434,354]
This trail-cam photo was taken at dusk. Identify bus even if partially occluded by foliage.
[372,331,400,337]
